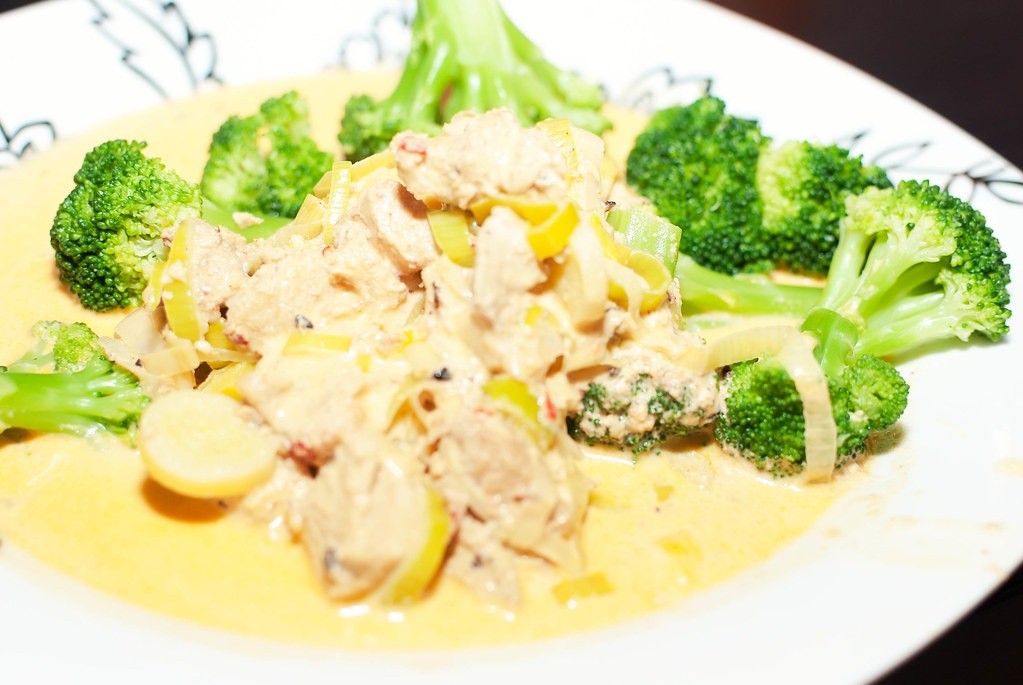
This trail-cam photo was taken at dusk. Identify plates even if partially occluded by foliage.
[3,1,1022,685]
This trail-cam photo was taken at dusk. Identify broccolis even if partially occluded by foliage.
[0,0,1010,473]
